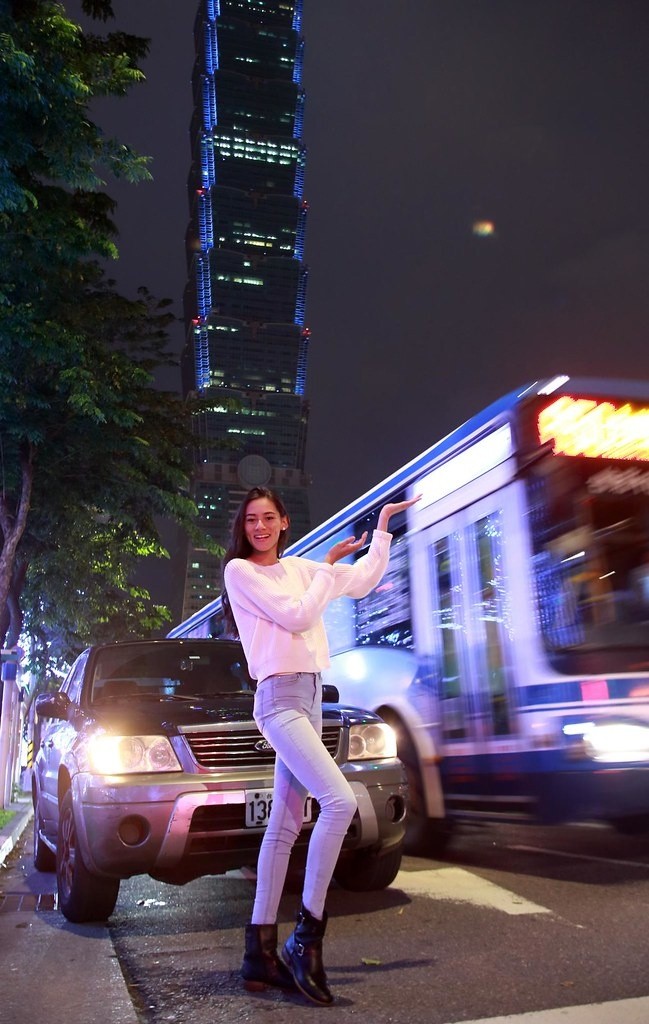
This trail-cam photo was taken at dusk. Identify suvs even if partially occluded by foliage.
[31,636,414,923]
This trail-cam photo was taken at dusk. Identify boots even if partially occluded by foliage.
[240,922,303,994]
[281,899,335,1005]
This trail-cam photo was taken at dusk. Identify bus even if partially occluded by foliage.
[170,373,649,851]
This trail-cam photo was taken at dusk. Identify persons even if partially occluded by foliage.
[221,484,425,1007]
